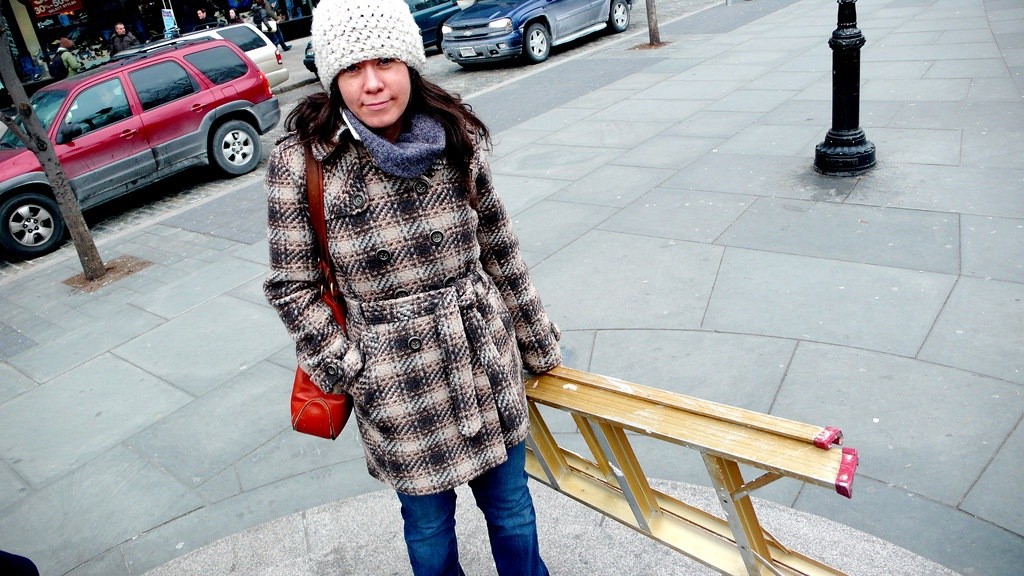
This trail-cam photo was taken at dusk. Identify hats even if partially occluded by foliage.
[310,0,428,98]
[60,38,72,48]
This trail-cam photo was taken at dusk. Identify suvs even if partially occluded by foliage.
[0,34,282,262]
[113,23,290,89]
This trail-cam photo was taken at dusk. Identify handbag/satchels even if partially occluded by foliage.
[290,143,354,440]
[260,17,277,32]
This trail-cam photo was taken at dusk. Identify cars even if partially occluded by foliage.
[440,0,637,73]
[303,0,461,77]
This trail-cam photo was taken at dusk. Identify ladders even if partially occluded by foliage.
[515,365,862,576]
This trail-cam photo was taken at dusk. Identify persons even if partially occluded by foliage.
[195,10,217,31]
[109,24,141,61]
[55,37,82,79]
[228,8,243,24]
[249,0,292,52]
[265,0,561,576]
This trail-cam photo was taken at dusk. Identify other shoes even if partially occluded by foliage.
[284,45,292,51]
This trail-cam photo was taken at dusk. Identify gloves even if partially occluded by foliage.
[268,27,272,32]
[80,62,84,66]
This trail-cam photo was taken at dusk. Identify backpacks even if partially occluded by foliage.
[48,50,70,79]
